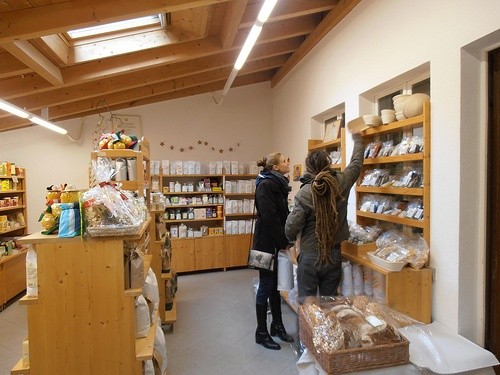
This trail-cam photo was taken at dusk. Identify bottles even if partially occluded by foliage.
[160,232,178,311]
[143,169,224,241]
[0,161,26,256]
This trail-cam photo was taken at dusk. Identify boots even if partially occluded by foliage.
[269,290,294,341]
[255,302,280,349]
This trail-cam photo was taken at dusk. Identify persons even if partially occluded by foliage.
[286,129,365,361]
[246,152,298,351]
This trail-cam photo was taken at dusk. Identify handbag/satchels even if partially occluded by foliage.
[248,249,275,271]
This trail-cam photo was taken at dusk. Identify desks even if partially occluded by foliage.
[296,322,496,375]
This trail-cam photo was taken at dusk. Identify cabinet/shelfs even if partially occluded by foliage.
[11,148,260,375]
[307,101,435,329]
[0,168,31,310]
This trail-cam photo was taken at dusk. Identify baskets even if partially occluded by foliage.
[298,306,412,375]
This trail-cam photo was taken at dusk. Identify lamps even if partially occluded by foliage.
[234,0,278,70]
[0,98,68,135]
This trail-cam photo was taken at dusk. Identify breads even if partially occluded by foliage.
[302,295,411,352]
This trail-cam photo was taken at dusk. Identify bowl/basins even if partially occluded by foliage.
[363,94,430,127]
